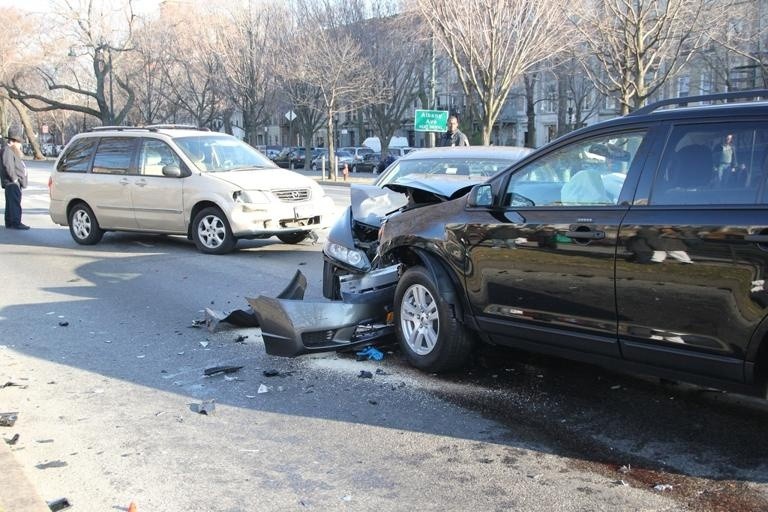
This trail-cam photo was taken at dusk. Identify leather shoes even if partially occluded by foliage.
[5,223,29,229]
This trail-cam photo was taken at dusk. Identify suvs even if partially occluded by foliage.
[48,124,338,255]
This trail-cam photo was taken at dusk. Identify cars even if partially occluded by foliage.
[389,147,414,159]
[258,146,377,168]
[310,150,355,172]
[350,153,397,175]
[377,89,768,400]
[322,147,566,304]
[20,142,47,156]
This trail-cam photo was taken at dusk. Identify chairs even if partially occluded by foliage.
[664,143,715,191]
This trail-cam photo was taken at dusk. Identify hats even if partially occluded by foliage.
[4,126,25,142]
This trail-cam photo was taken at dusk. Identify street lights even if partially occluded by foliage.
[68,44,115,126]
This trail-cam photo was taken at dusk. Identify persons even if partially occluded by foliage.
[712,135,737,186]
[1,125,30,230]
[440,117,470,148]
